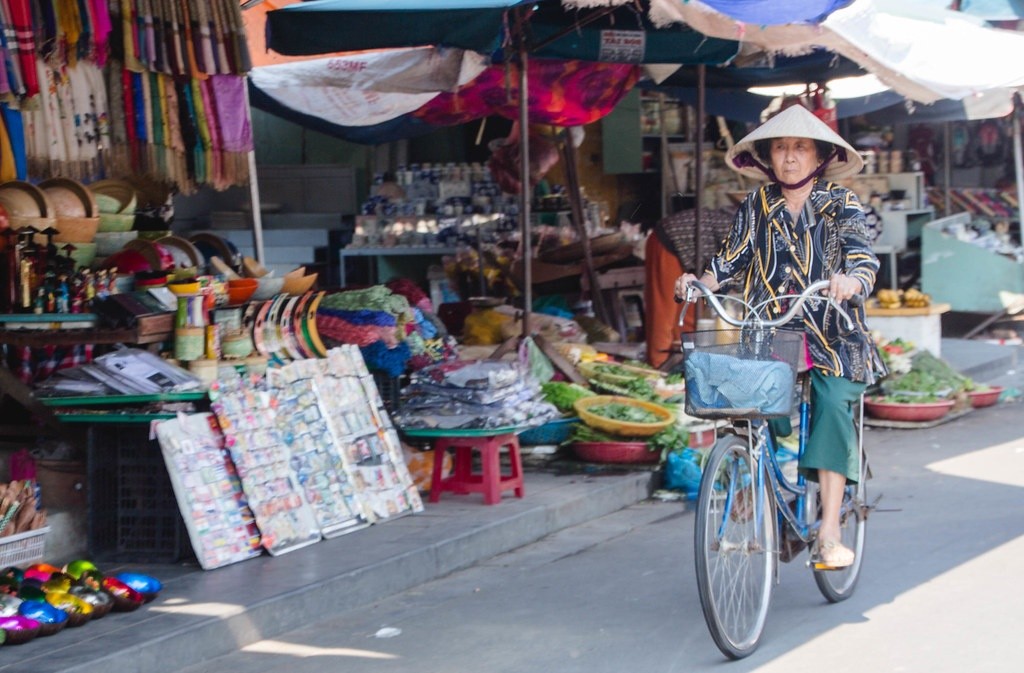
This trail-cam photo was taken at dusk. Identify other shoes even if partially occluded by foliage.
[729,483,758,522]
[814,540,853,568]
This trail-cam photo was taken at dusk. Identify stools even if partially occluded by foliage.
[432,437,523,505]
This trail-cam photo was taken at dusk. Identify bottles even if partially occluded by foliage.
[189,359,217,382]
[175,328,205,360]
[220,334,253,359]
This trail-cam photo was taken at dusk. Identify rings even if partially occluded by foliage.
[674,286,680,291]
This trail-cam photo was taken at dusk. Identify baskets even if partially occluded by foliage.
[522,360,685,466]
[680,327,803,419]
[962,385,1005,408]
[865,395,956,420]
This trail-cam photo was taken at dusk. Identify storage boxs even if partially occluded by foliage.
[1,526,53,572]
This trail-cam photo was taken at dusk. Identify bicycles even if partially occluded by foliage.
[674,282,903,661]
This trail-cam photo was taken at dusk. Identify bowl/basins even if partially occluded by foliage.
[1,178,318,303]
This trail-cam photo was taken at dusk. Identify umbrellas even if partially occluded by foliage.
[265,0,1024,385]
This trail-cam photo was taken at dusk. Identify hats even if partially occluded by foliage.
[725,104,863,182]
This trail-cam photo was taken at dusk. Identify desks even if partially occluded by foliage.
[338,239,474,294]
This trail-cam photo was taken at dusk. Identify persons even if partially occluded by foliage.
[672,104,891,568]
